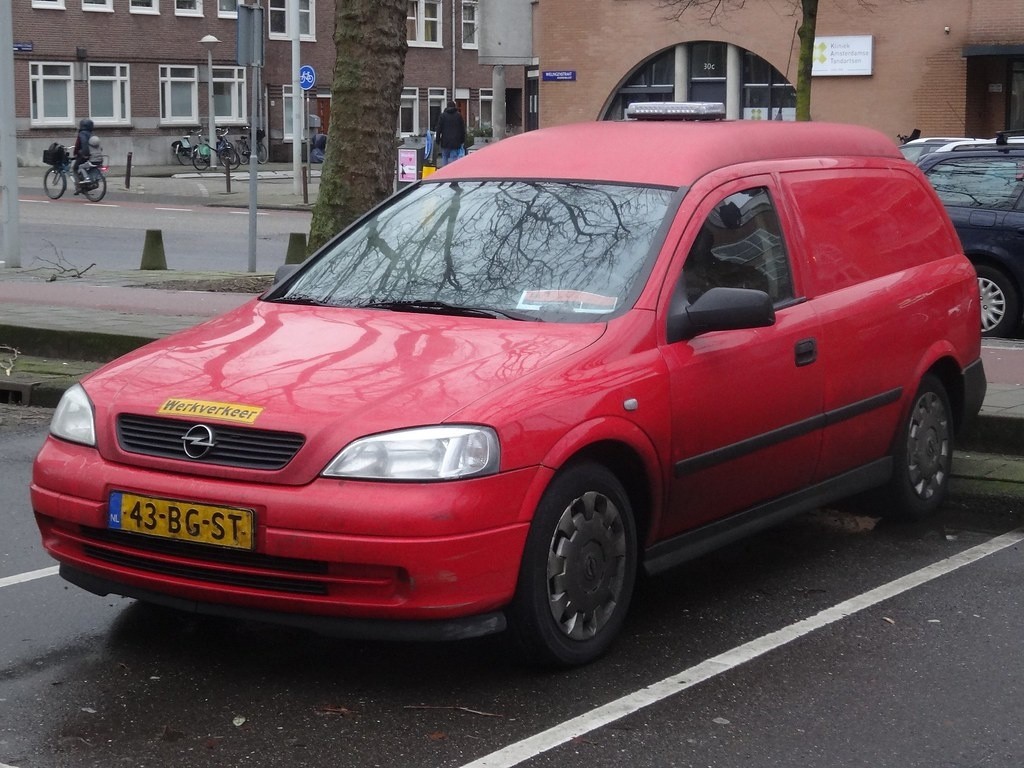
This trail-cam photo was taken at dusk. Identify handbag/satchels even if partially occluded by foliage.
[458,144,465,159]
[47,143,65,164]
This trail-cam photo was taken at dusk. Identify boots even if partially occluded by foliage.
[74,182,82,195]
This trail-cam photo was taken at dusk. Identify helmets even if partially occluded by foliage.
[88,136,100,146]
[78,119,93,132]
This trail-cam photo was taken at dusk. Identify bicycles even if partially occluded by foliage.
[41,144,109,202]
[173,127,270,171]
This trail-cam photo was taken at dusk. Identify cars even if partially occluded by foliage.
[29,120,989,653]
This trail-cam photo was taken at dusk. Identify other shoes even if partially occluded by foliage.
[78,179,92,184]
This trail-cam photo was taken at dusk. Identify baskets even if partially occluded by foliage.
[43,150,64,165]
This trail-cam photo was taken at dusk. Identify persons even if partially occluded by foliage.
[72,118,103,195]
[435,101,465,167]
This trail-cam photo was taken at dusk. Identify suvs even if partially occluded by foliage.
[895,133,1024,341]
